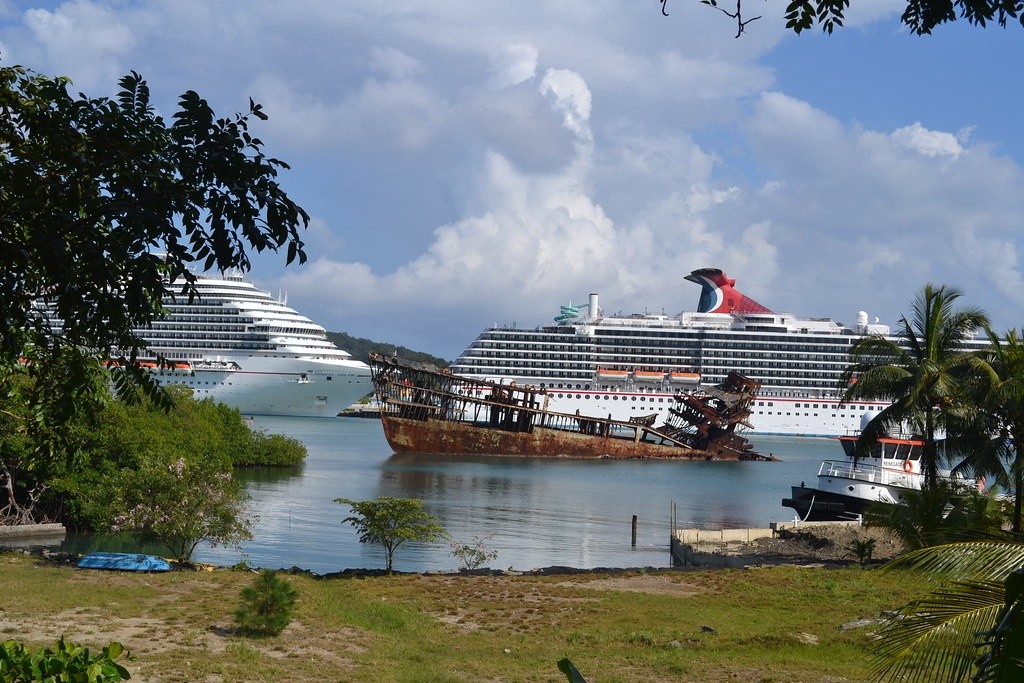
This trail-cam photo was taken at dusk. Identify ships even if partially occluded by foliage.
[13,253,379,417]
[401,267,1024,441]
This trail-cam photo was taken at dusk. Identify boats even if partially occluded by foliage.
[367,351,783,462]
[781,411,985,522]
[77,551,175,572]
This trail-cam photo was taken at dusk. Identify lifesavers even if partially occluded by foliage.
[903,460,913,473]
